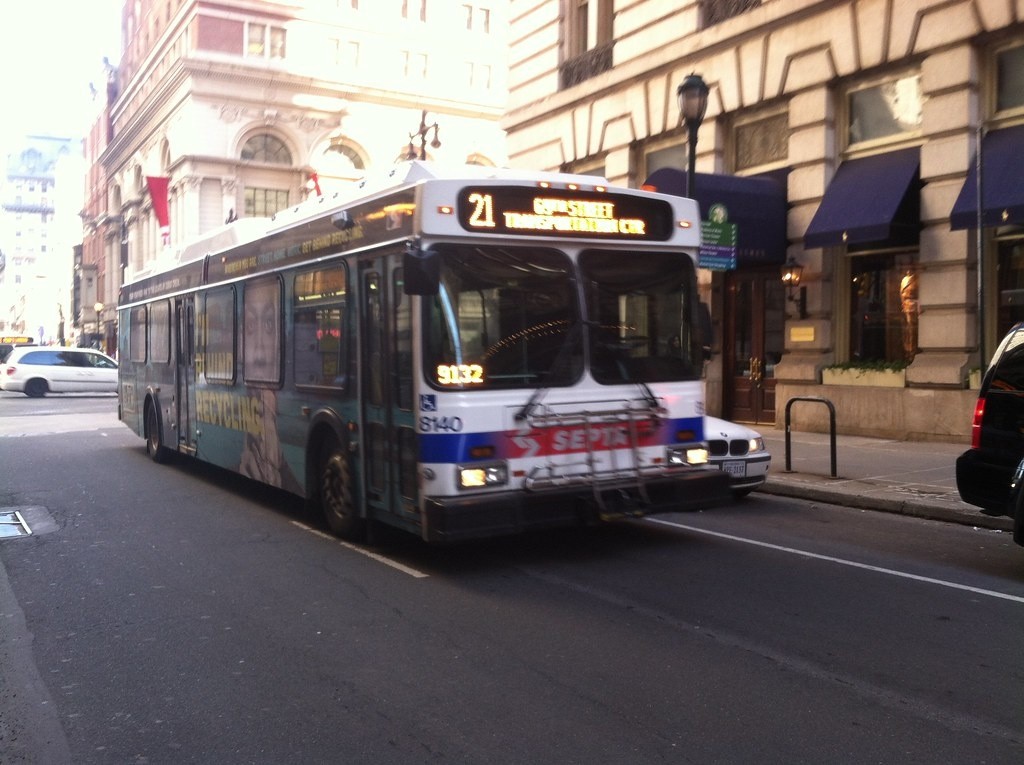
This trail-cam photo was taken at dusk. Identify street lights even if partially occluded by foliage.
[406,110,441,161]
[93,303,103,367]
[676,73,710,199]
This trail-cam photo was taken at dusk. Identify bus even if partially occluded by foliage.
[116,161,733,544]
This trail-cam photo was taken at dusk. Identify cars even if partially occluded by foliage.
[682,415,771,506]
[956,320,1024,550]
[0,343,38,364]
[0,347,119,397]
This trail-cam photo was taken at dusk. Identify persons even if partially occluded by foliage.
[75,335,103,365]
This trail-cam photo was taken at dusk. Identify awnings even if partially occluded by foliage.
[804,146,920,249]
[950,124,1024,230]
[642,167,787,265]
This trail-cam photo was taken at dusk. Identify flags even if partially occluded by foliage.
[146,176,170,245]
